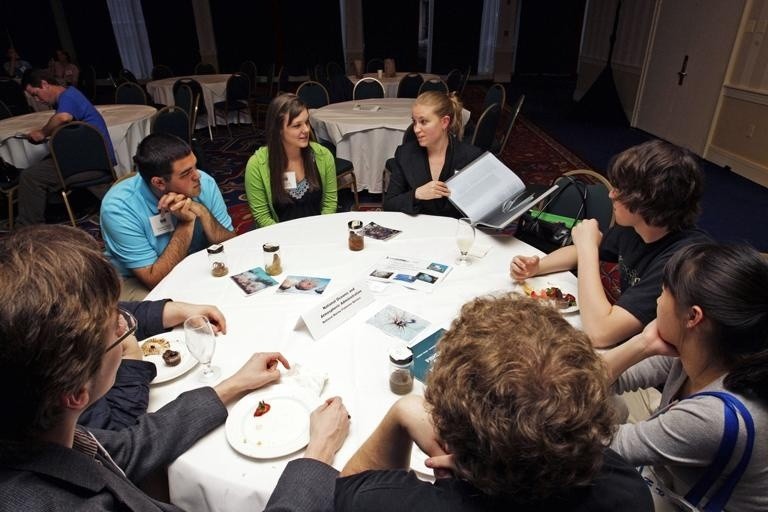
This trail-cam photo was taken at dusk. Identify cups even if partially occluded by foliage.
[378,70,383,81]
[354,60,365,79]
[384,61,395,78]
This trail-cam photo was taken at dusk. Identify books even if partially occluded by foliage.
[441,149,559,230]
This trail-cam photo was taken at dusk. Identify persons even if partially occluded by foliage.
[381,86,481,217]
[508,138,715,349]
[243,90,338,227]
[334,290,656,512]
[99,129,236,301]
[0,223,351,511]
[600,239,767,512]
[14,65,117,234]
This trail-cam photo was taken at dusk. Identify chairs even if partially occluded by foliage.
[0,54,533,232]
[520,168,618,271]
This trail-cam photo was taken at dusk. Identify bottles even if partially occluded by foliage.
[348,220,365,252]
[208,243,229,278]
[262,241,284,277]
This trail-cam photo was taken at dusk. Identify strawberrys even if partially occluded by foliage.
[254,402,269,416]
[565,294,574,305]
[547,285,562,299]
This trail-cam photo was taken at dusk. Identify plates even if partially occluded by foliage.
[225,382,326,459]
[514,277,579,314]
[135,330,202,386]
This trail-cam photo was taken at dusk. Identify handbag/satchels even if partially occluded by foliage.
[514,174,587,254]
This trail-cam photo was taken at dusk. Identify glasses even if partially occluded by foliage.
[106,308,138,352]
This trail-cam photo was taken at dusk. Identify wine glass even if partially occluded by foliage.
[455,218,476,266]
[183,315,223,382]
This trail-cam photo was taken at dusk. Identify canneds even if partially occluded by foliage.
[263,244,281,276]
[347,221,364,250]
[208,245,227,276]
